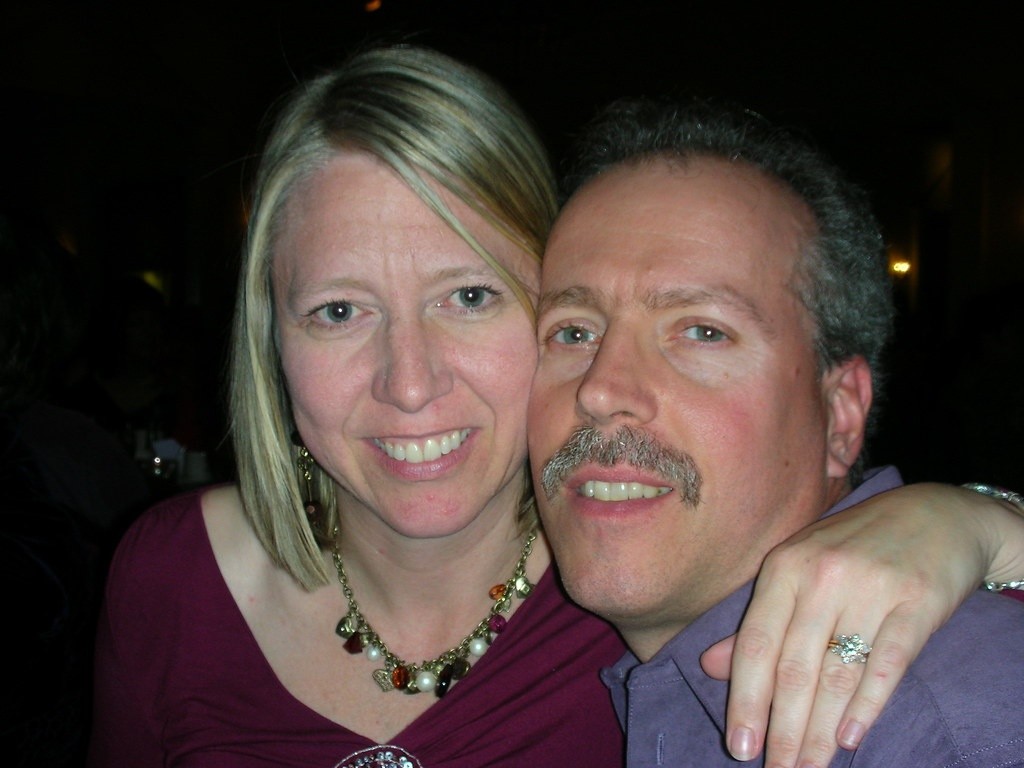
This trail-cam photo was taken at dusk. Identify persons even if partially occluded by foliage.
[95,44,1024,768]
[526,104,1024,768]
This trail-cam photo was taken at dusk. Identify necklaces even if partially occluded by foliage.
[329,527,535,704]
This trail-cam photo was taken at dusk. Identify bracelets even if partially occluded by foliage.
[958,483,1024,593]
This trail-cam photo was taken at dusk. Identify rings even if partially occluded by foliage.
[825,633,873,665]
